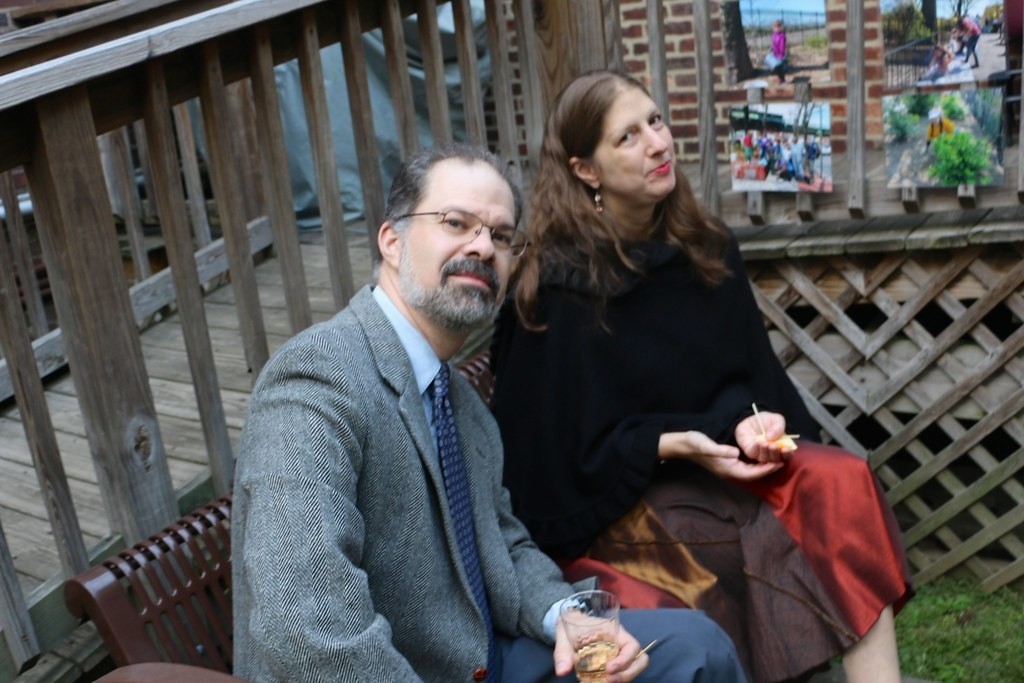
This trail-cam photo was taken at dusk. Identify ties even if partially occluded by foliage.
[425,357,503,683]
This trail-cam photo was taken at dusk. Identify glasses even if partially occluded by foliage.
[384,201,531,260]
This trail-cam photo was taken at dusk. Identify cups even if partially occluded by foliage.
[560,589,621,682]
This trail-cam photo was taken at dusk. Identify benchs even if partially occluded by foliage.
[66,349,498,676]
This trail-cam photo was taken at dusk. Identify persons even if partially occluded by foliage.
[490,68,915,683]
[929,10,990,74]
[236,143,749,683]
[743,124,821,185]
[924,107,955,156]
[767,18,787,66]
